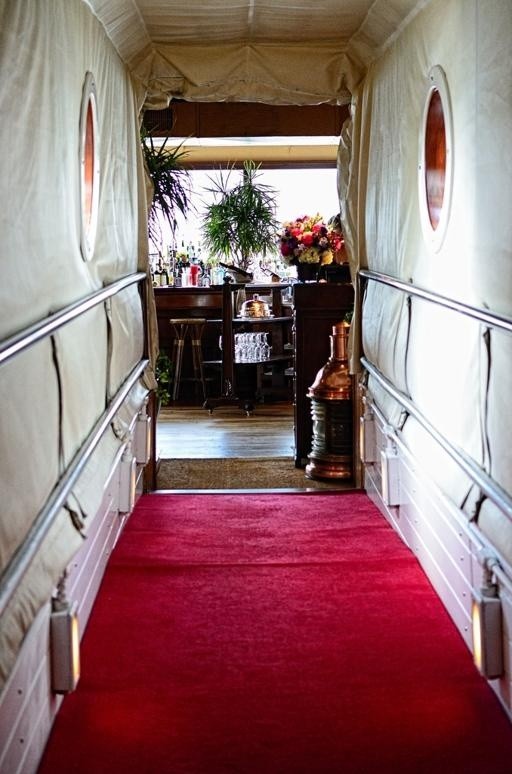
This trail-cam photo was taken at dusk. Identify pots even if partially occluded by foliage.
[236,293,275,319]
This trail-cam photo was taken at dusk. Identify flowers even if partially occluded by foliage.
[277,213,343,266]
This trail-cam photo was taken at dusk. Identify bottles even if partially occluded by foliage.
[150,238,225,287]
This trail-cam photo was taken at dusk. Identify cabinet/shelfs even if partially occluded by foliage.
[202,317,293,365]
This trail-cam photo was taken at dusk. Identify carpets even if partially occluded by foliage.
[155,456,351,489]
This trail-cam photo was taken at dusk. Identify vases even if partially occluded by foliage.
[296,263,319,282]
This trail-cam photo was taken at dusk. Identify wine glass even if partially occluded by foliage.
[218,332,271,359]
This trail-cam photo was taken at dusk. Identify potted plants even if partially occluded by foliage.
[204,160,269,282]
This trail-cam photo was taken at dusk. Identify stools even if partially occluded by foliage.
[169,317,209,400]
